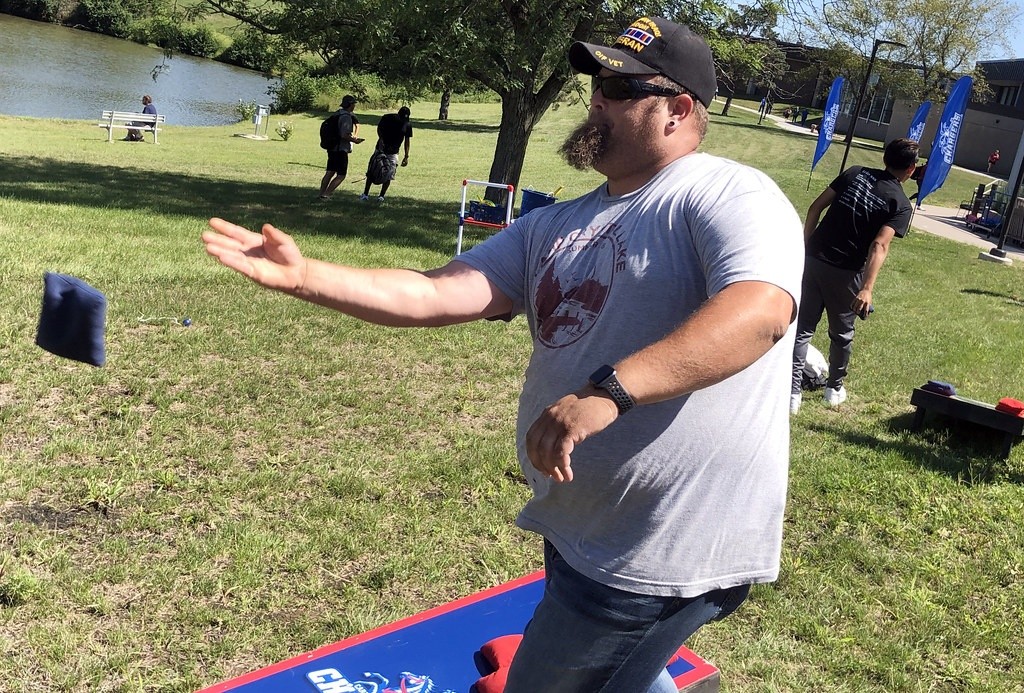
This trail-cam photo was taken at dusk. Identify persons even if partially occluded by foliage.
[909,140,934,211]
[784,106,809,127]
[714,86,718,100]
[815,117,823,134]
[203,18,806,693]
[439,89,451,120]
[356,106,413,203]
[987,149,1000,175]
[317,95,365,201]
[790,138,920,416]
[122,95,157,141]
[758,94,774,114]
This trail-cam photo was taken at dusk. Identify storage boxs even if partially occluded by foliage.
[469,200,505,224]
[192,568,722,693]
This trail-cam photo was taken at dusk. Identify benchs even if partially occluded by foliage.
[98,110,165,144]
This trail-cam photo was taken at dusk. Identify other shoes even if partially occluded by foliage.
[133,135,145,142]
[123,136,134,141]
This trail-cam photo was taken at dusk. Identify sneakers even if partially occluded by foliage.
[790,392,802,414]
[360,193,368,201]
[825,386,846,405]
[378,197,385,202]
[317,195,332,202]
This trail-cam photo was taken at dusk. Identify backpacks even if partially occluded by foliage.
[320,113,351,149]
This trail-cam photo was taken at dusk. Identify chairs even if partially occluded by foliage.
[456,179,513,255]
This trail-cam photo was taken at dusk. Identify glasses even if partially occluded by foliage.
[591,74,698,100]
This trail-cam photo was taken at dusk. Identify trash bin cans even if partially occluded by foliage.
[520,185,558,220]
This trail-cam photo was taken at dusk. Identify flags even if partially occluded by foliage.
[905,102,932,144]
[916,76,973,207]
[811,77,843,169]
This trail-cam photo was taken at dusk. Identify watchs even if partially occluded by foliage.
[587,363,638,415]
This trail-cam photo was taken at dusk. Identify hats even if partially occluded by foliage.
[568,17,717,110]
[340,95,357,108]
[398,106,410,122]
